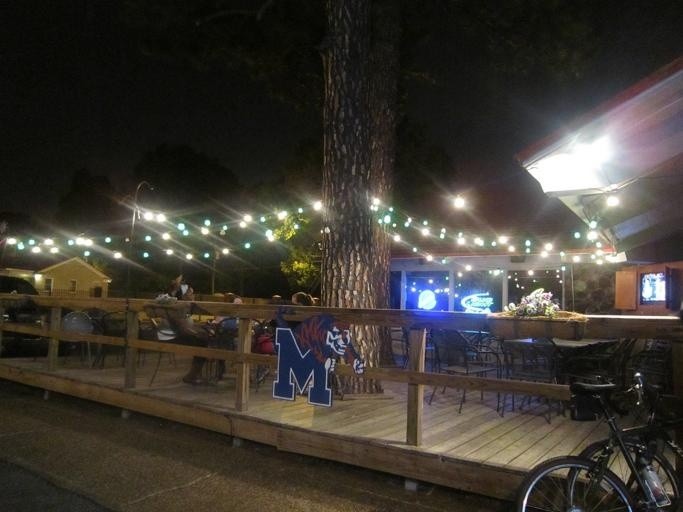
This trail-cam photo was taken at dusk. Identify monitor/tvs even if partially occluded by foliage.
[639,271,667,304]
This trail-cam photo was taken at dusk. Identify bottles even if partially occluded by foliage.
[645,466,671,507]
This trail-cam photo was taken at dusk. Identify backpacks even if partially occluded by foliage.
[255,322,273,353]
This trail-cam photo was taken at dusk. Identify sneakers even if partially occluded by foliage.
[256,366,269,383]
[183,376,223,385]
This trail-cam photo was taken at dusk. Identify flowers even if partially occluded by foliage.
[503,287,560,320]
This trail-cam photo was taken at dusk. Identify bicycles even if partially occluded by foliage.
[516,369,683,512]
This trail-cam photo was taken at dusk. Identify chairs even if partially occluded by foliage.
[83,303,278,393]
[385,325,672,421]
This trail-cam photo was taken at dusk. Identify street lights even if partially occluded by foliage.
[124,181,154,336]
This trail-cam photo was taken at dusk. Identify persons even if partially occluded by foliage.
[148,273,320,387]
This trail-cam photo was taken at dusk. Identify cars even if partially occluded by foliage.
[0,276,78,357]
